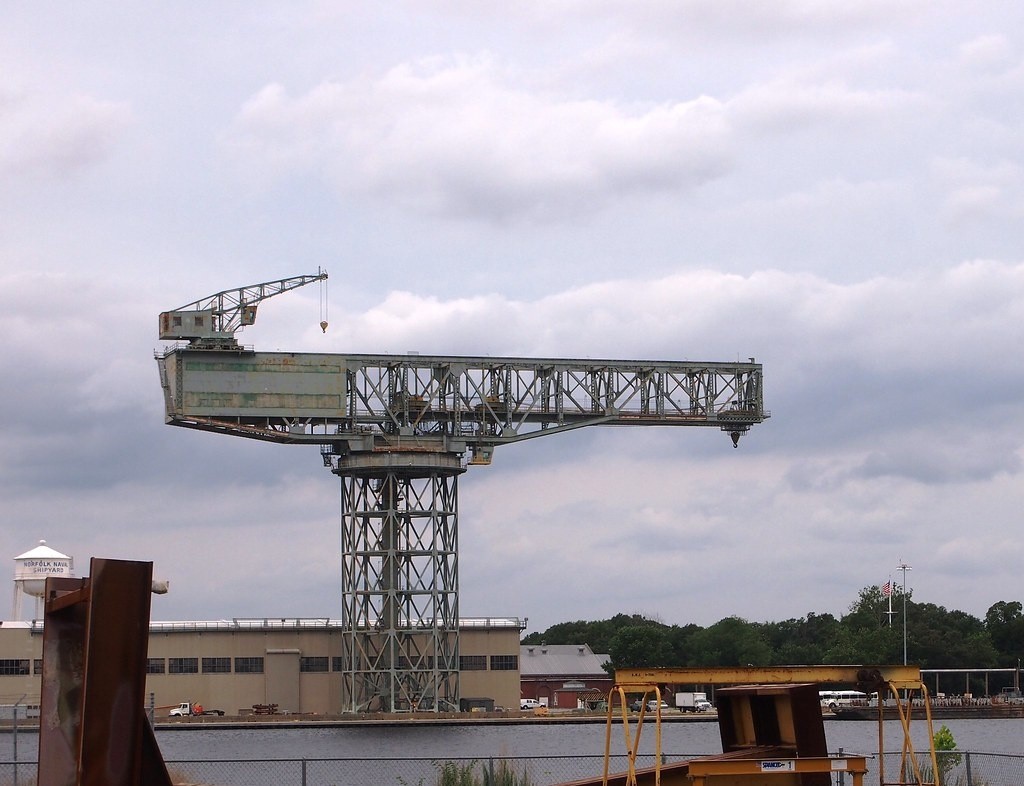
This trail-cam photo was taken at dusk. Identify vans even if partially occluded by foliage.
[647,699,669,711]
[520,698,547,709]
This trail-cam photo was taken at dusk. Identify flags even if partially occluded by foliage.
[882,580,890,596]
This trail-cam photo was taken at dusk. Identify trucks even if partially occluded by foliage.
[675,693,713,713]
[169,702,225,716]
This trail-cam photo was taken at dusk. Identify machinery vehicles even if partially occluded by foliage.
[159,266,330,350]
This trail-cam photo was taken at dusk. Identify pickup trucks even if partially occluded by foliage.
[629,701,651,712]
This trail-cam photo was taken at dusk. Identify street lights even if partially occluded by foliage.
[896,563,913,705]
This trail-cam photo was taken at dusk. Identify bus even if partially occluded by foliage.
[819,690,879,709]
